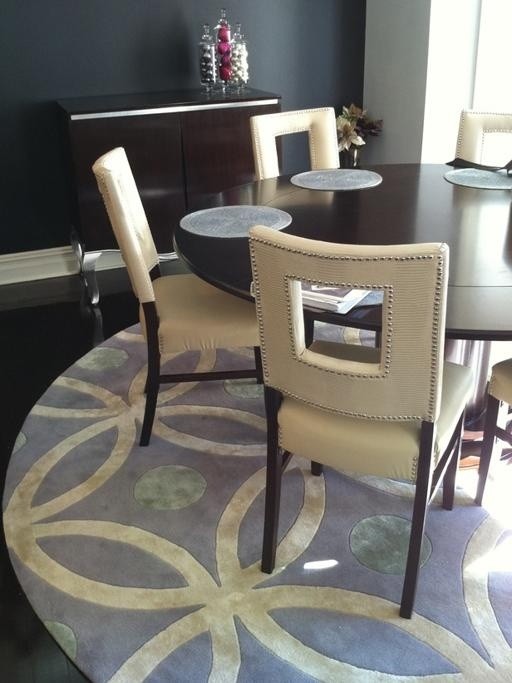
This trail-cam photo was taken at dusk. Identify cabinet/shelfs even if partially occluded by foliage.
[57,84,282,271]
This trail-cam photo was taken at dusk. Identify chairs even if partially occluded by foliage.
[455,110,511,164]
[474,359,512,507]
[244,226,473,616]
[95,146,263,446]
[250,105,340,182]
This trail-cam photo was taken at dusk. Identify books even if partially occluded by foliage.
[250,278,372,316]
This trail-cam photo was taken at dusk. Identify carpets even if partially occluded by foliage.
[0,323,512,681]
